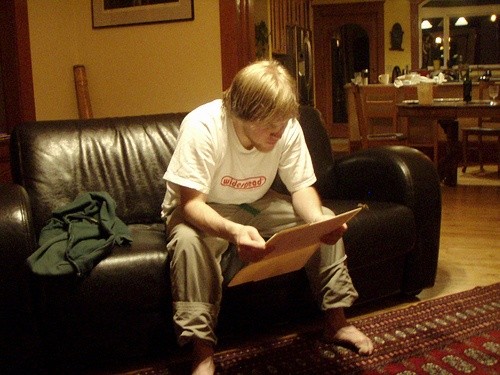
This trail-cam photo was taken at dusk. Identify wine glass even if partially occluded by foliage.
[488,85,499,105]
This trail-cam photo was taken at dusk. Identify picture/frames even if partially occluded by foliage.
[90,0,195,30]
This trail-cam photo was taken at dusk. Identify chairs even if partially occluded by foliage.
[352,85,500,175]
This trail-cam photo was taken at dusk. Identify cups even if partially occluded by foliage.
[378,74,389,84]
[463,68,472,102]
[354,69,369,85]
[433,60,440,71]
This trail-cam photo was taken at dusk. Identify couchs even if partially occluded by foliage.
[0,107,442,375]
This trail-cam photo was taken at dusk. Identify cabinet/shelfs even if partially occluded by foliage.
[220,0,312,93]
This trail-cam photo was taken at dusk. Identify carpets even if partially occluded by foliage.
[128,281,500,375]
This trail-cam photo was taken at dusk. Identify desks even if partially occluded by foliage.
[396,97,500,186]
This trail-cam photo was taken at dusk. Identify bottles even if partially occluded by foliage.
[457,54,464,80]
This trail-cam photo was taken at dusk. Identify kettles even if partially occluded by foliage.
[392,66,402,83]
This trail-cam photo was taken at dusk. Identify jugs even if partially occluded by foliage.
[417,81,434,105]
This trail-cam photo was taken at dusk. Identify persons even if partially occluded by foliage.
[160,60,373,375]
